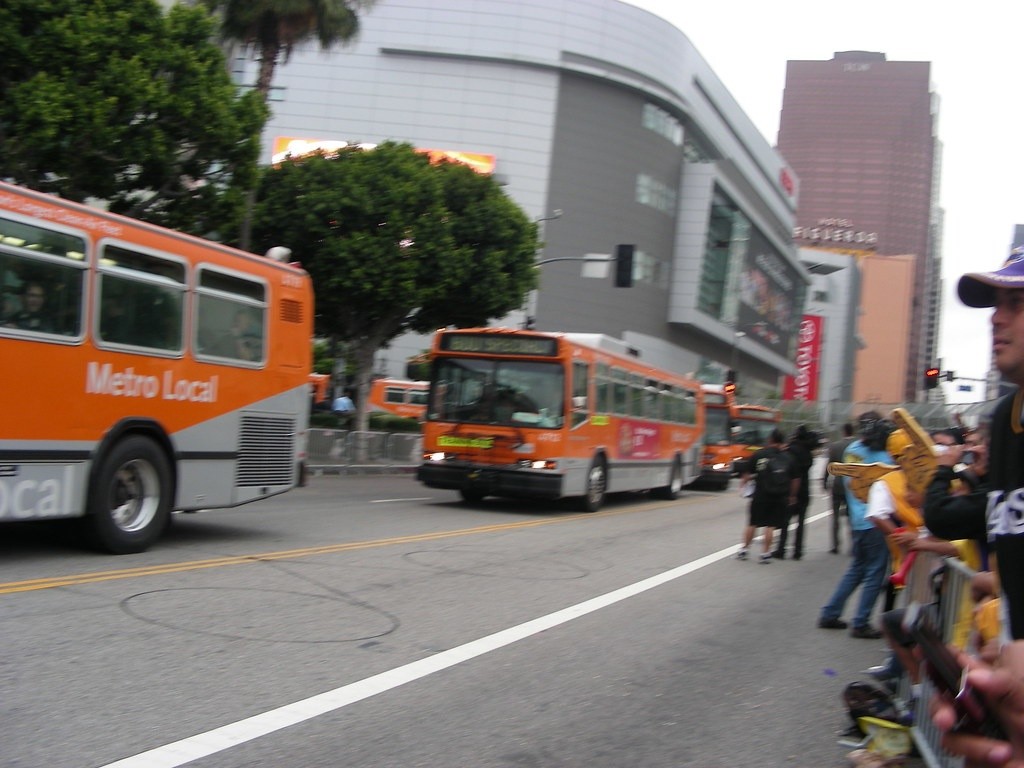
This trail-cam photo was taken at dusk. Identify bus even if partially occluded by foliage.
[405,326,707,513]
[370,378,430,423]
[0,177,316,558]
[696,383,781,492]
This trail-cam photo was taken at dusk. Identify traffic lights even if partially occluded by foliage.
[924,366,939,389]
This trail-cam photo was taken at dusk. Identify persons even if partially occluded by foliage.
[0,283,160,350]
[730,246,1024,768]
[467,377,557,426]
[330,387,355,412]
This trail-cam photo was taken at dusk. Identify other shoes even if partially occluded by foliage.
[772,551,783,559]
[758,553,771,561]
[852,624,884,640]
[738,549,747,557]
[820,617,849,631]
[794,553,801,559]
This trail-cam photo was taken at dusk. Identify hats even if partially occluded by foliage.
[958,245,1024,308]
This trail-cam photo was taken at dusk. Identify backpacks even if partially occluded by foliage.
[764,448,792,496]
